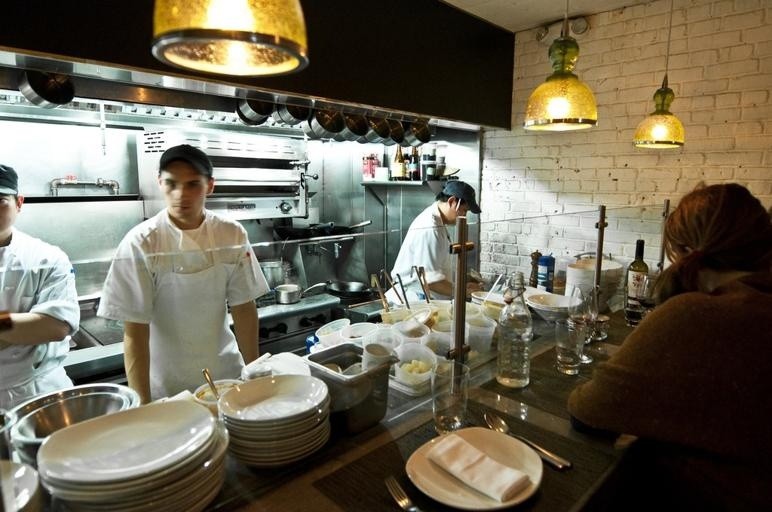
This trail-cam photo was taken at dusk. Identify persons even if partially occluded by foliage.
[386,179,486,311]
[565,181,772,512]
[94,146,273,408]
[1,165,81,412]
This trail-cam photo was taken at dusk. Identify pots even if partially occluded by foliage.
[326,280,387,299]
[259,261,290,290]
[274,218,375,240]
[16,72,77,108]
[274,280,325,304]
[235,101,436,146]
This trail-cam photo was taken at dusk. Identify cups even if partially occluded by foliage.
[429,361,471,430]
[361,344,391,372]
[555,316,586,377]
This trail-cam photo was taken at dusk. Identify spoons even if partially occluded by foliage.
[483,410,574,469]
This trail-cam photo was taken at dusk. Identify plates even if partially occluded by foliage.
[38,400,229,510]
[218,374,334,468]
[403,427,544,509]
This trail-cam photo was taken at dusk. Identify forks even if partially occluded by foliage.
[382,476,420,512]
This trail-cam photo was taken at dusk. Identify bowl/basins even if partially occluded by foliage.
[530,293,584,318]
[3,379,140,444]
[472,288,504,307]
[314,305,499,386]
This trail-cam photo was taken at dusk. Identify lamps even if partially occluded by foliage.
[148,0,310,78]
[522,1,597,133]
[632,1,688,149]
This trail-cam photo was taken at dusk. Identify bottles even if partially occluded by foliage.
[528,250,542,286]
[555,249,578,286]
[623,237,649,325]
[538,250,555,289]
[495,271,533,390]
[393,144,419,181]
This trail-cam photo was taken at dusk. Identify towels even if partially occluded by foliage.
[425,431,530,502]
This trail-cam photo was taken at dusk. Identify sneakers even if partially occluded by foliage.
[160,145,212,175]
[442,180,482,214]
[1,165,18,194]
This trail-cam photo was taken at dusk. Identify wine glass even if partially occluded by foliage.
[568,282,599,365]
